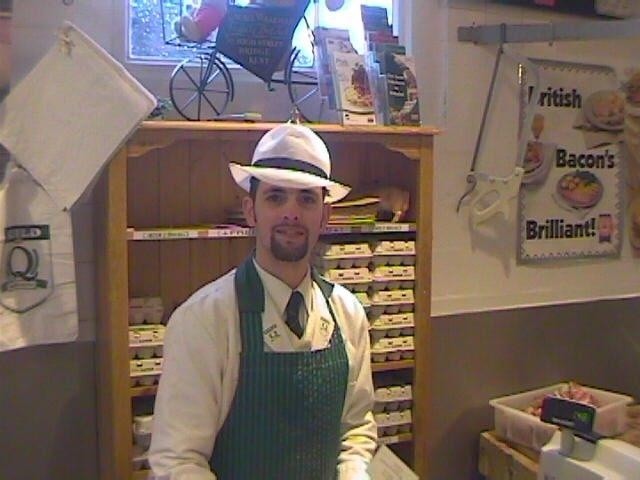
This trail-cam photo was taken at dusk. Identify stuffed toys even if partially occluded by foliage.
[174,0,227,42]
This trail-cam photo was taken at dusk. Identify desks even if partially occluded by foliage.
[476,400,639,480]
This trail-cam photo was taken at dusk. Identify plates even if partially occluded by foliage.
[523,140,549,184]
[557,171,603,208]
[583,91,625,130]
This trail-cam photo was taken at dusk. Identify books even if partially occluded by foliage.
[0,23,157,212]
[360,5,419,126]
[314,26,377,126]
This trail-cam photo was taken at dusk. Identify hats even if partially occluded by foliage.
[227,124,352,204]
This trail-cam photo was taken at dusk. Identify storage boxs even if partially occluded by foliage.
[488,378,634,450]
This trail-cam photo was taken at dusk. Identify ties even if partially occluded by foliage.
[285,291,307,338]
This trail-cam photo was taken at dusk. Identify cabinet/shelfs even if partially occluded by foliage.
[94,119,445,480]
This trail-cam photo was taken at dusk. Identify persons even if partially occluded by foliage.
[148,124,379,480]
